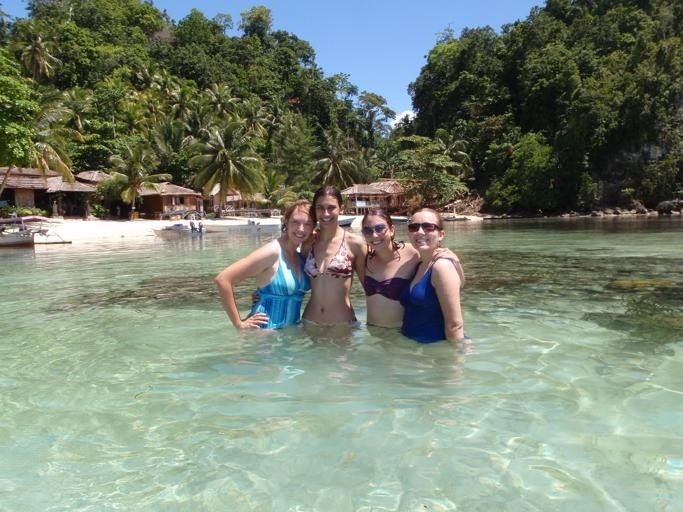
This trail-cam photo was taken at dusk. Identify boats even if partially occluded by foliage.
[0,215,73,244]
[152,223,207,232]
[244,216,357,227]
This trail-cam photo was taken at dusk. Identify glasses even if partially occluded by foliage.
[408,223,440,232]
[362,224,386,234]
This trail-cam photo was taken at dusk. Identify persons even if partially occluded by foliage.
[356,208,464,328]
[213,199,322,332]
[397,206,472,353]
[250,185,373,329]
